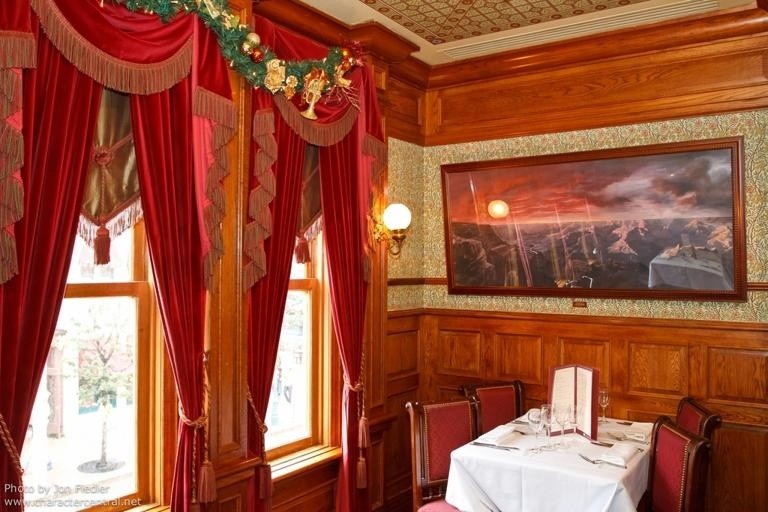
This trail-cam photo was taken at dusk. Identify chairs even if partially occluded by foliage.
[405,395,479,511]
[647,414,709,512]
[673,397,723,454]
[466,379,523,436]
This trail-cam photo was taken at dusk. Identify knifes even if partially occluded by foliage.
[467,441,520,453]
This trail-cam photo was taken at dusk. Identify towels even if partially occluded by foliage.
[624,421,653,441]
[600,441,638,467]
[518,409,547,423]
[473,424,515,445]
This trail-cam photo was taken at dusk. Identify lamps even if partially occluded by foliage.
[372,202,413,256]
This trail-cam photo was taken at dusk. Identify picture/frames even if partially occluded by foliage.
[440,134,748,303]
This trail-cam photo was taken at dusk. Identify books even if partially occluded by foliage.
[547,364,600,442]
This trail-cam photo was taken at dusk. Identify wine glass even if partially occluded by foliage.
[597,385,610,423]
[525,403,582,454]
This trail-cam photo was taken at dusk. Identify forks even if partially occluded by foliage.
[577,455,627,469]
